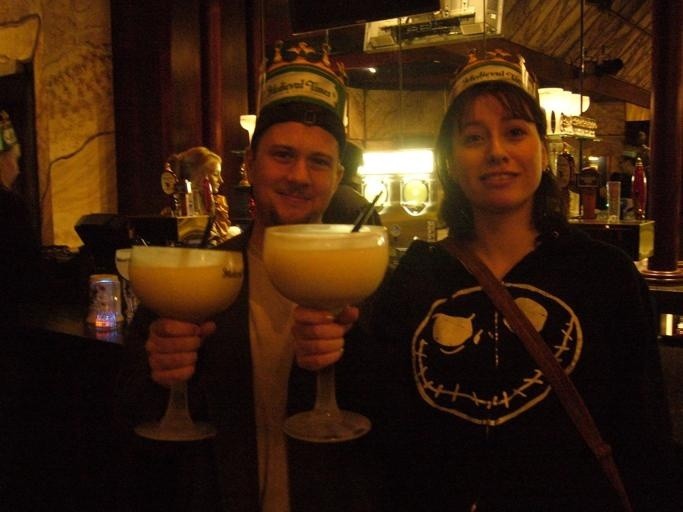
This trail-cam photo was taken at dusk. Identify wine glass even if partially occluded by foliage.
[125,241,245,442]
[259,221,391,447]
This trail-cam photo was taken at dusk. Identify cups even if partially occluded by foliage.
[605,181,621,218]
[582,189,596,218]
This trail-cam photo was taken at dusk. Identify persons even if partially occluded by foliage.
[100,99,392,511]
[159,145,232,249]
[1,108,93,512]
[554,131,649,223]
[285,83,670,512]
